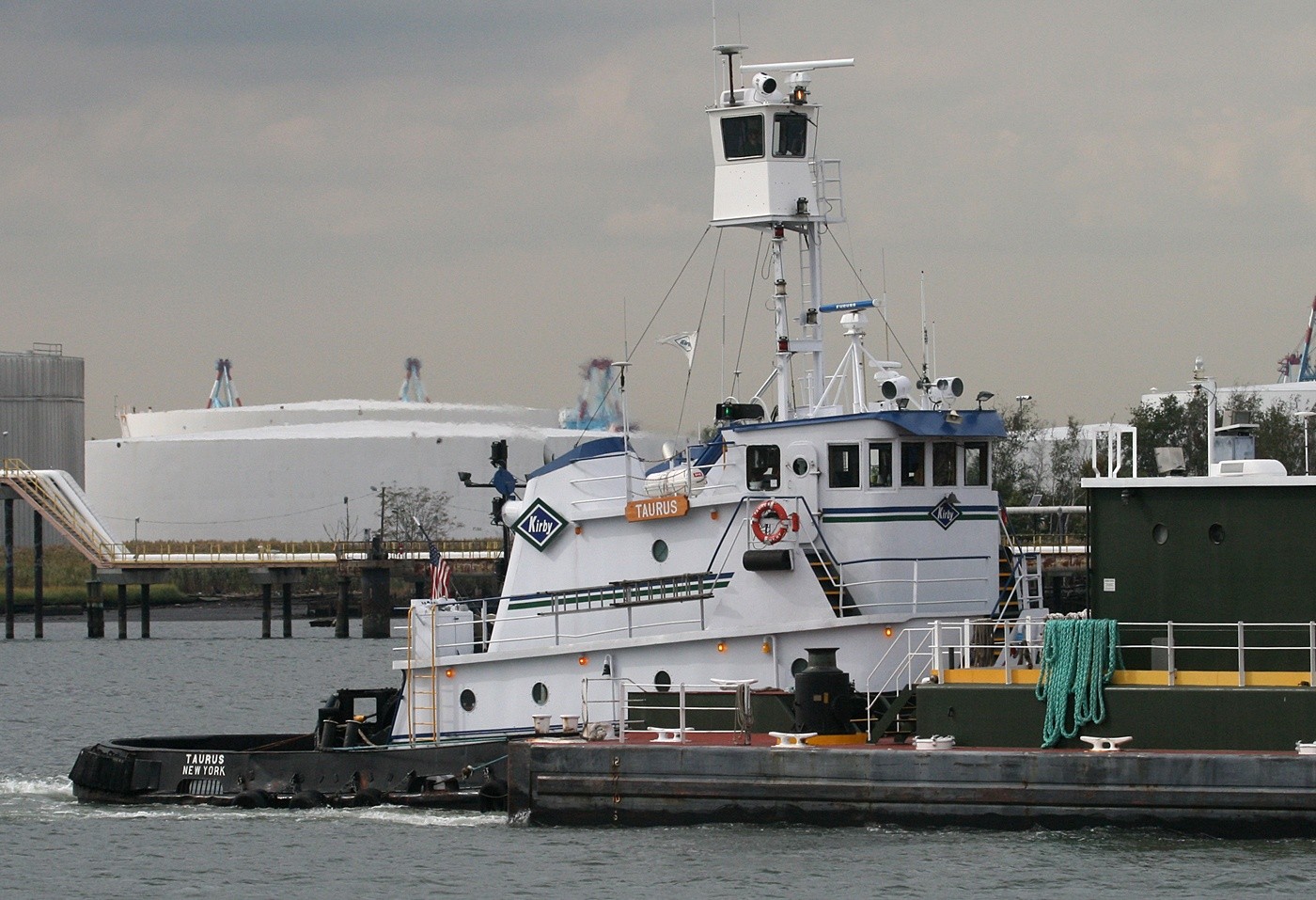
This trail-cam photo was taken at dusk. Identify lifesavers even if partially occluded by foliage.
[750,501,791,545]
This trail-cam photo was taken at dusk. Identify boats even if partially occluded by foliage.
[68,0,1316,840]
